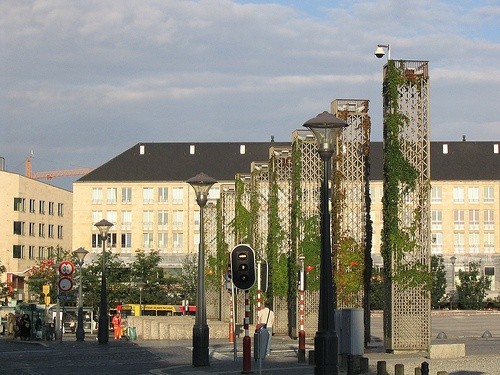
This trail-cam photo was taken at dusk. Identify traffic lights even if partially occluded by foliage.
[230,244,256,290]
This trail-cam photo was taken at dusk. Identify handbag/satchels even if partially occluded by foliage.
[255,323,267,333]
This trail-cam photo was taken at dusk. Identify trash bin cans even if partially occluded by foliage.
[128,326,136,340]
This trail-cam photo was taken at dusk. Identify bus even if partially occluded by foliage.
[116,303,197,339]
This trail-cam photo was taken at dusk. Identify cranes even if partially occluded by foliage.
[25,156,96,180]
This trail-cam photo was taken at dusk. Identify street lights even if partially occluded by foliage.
[302,109,350,375]
[450,256,457,294]
[73,247,90,341]
[94,218,115,343]
[186,171,219,368]
[137,280,147,304]
